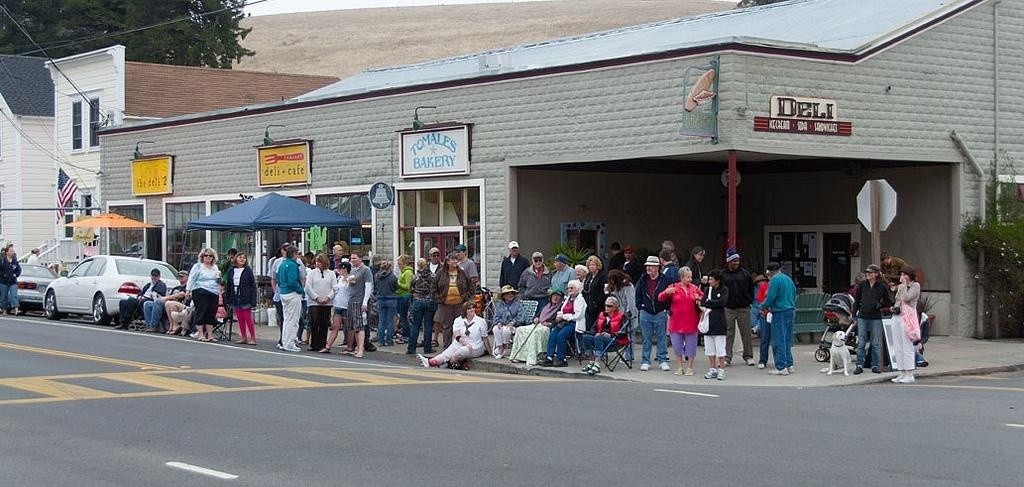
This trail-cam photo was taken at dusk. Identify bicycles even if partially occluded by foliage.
[368,284,495,333]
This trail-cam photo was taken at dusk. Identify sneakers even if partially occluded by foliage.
[899,377,915,383]
[114,323,260,347]
[891,376,901,384]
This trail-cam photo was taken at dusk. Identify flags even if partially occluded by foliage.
[55,166,80,224]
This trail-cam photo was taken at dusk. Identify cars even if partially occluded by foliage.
[17,263,67,316]
[41,253,184,324]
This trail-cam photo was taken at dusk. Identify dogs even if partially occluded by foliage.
[819,331,852,376]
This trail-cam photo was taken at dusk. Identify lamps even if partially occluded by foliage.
[264,123,285,147]
[412,104,437,131]
[134,140,156,160]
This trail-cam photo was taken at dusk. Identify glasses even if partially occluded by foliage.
[604,304,614,307]
[467,306,476,310]
[534,260,543,262]
[152,273,158,277]
[203,254,212,257]
[566,286,576,290]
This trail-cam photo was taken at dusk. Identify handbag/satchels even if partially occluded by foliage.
[697,286,713,334]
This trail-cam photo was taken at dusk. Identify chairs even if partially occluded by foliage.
[578,310,637,371]
[206,305,233,342]
[520,299,539,326]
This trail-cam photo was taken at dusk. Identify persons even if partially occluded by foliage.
[0,241,23,318]
[634,240,798,382]
[117,241,374,359]
[369,241,639,375]
[27,248,44,266]
[846,250,930,382]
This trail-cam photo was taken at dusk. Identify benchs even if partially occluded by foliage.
[792,291,833,342]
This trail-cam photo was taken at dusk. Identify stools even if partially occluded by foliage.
[128,305,145,331]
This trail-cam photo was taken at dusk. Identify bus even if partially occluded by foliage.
[329,224,371,256]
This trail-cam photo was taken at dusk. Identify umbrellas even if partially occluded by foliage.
[60,212,165,255]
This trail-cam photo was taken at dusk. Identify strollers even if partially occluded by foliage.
[814,292,859,362]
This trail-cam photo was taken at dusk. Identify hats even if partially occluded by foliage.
[454,244,465,252]
[767,261,781,271]
[498,284,518,298]
[552,255,568,265]
[549,287,565,301]
[507,241,519,250]
[623,244,633,253]
[429,247,440,254]
[725,247,741,263]
[864,264,880,274]
[899,265,916,281]
[753,274,768,284]
[176,270,188,277]
[643,255,661,266]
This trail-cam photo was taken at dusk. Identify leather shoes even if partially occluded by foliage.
[853,367,863,374]
[872,367,882,374]
